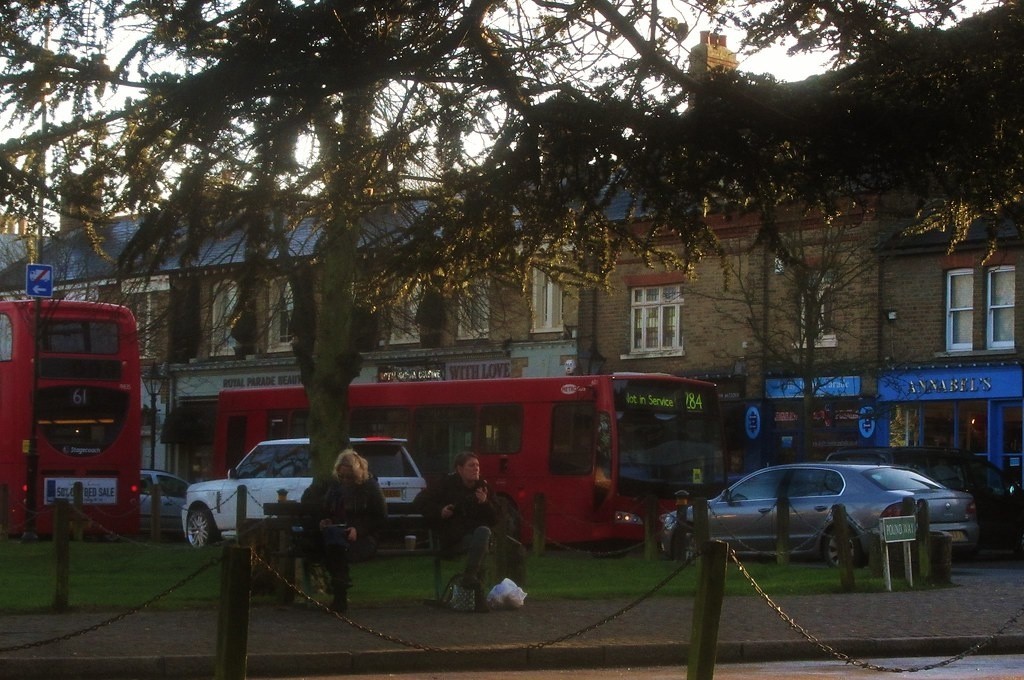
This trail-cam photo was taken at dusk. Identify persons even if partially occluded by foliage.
[425,451,504,613]
[301,448,388,612]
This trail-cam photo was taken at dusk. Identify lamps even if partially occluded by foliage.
[886,357,895,370]
[882,307,898,321]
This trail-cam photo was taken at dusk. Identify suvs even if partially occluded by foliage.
[827,446,1024,561]
[182,434,428,548]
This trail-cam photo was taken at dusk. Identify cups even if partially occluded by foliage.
[405,536,416,550]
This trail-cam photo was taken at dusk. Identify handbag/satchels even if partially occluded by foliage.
[488,577,528,611]
[445,583,476,612]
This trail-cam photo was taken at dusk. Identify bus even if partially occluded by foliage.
[214,372,727,558]
[0,299,141,534]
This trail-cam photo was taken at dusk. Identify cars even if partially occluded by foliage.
[98,470,190,542]
[661,462,979,568]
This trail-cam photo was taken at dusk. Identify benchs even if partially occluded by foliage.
[263,502,462,609]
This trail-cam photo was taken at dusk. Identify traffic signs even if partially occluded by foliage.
[25,262,54,299]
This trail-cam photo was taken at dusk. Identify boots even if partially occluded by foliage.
[475,590,490,613]
[326,545,349,612]
[460,564,478,593]
